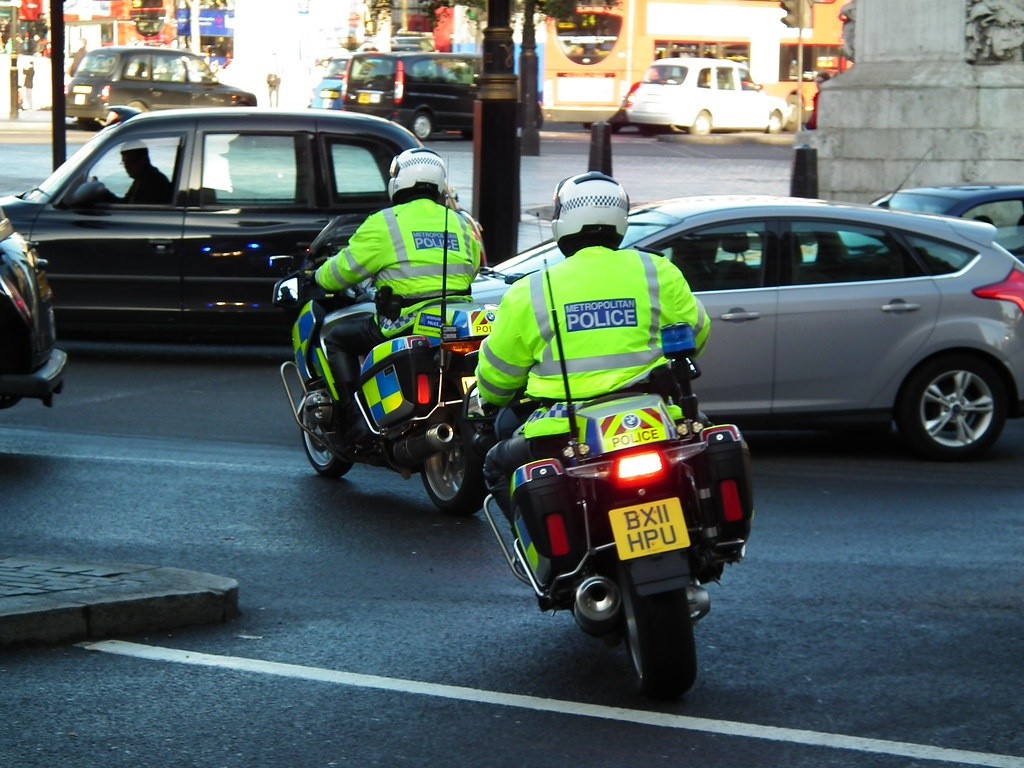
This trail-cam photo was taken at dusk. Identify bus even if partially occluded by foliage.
[18,0,177,56]
[542,0,853,129]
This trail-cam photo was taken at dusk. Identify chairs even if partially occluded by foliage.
[713,232,754,290]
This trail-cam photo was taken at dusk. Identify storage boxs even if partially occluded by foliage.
[689,423,754,543]
[509,458,583,591]
[359,335,437,427]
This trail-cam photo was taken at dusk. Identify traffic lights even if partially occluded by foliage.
[780,1,802,27]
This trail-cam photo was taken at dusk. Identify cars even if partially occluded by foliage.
[0,205,68,411]
[748,184,1024,264]
[307,55,381,112]
[471,193,1024,463]
[0,106,461,354]
[65,47,257,128]
[625,56,789,136]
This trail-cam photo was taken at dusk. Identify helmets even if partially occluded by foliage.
[387,147,446,203]
[552,171,630,257]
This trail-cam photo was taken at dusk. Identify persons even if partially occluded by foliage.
[18,60,35,109]
[473,172,713,584]
[307,147,481,444]
[104,140,172,206]
[806,71,830,129]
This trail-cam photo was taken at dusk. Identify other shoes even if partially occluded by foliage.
[344,414,368,441]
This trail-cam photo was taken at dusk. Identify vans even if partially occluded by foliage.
[343,52,482,142]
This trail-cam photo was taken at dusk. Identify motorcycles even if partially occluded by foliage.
[269,210,488,517]
[465,332,754,701]
